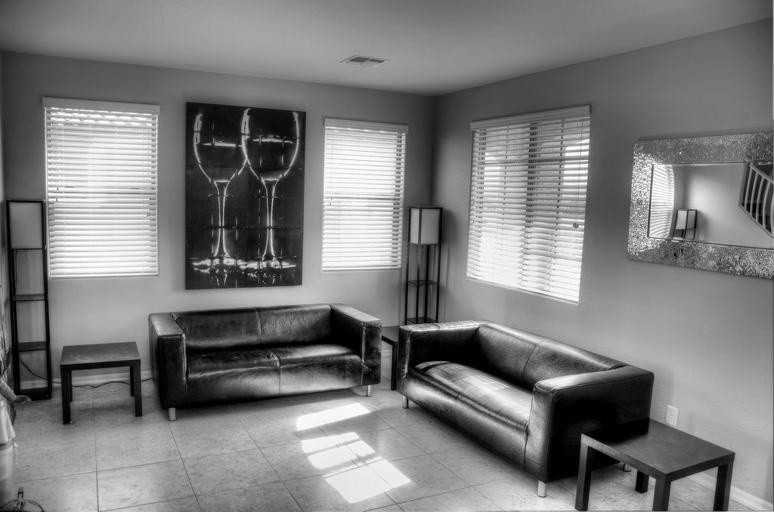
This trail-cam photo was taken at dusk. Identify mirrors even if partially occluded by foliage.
[625,130,774,281]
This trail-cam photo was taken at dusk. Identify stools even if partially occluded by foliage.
[58,341,143,426]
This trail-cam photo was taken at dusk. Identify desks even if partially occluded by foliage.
[379,325,399,392]
[571,415,736,512]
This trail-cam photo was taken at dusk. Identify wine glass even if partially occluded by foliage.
[239,108,300,272]
[191,110,249,274]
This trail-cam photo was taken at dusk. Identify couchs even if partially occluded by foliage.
[147,302,383,422]
[394,318,655,499]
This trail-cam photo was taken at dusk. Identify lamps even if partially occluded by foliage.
[1,198,54,402]
[671,208,698,241]
[403,205,443,325]
